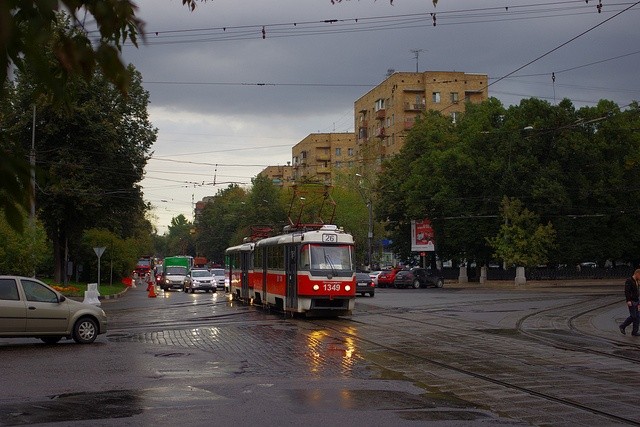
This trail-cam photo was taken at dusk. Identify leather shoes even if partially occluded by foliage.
[619,325,626,335]
[631,330,640,336]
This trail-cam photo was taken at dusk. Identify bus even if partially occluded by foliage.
[174,255,195,267]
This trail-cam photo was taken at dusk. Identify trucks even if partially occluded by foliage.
[136,255,154,276]
[165,256,188,290]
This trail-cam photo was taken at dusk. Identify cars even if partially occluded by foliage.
[156,266,164,284]
[369,270,382,286]
[1,274,108,344]
[355,272,375,297]
[394,269,444,288]
[184,269,218,292]
[378,268,402,288]
[211,268,225,287]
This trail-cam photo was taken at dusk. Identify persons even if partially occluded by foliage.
[619,269,640,336]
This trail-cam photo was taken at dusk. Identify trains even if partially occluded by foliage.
[224,224,357,317]
